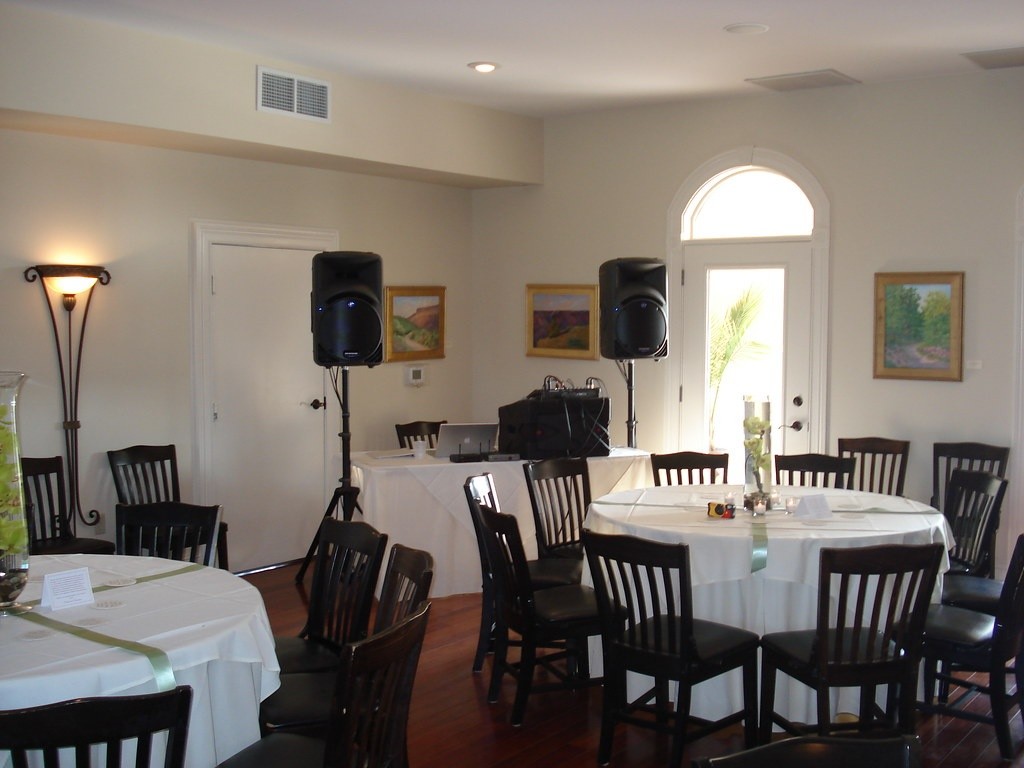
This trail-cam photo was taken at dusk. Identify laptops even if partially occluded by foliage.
[425,422,499,458]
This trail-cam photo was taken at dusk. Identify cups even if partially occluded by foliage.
[752,498,767,518]
[412,441,428,461]
[786,497,801,513]
[724,492,736,506]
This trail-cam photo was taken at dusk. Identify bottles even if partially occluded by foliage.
[0,370,32,616]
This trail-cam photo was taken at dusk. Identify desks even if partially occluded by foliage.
[581,482,953,731]
[0,554,281,768]
[332,444,651,598]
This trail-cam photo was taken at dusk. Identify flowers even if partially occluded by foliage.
[742,415,772,511]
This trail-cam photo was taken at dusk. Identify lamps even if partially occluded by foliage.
[20,263,114,529]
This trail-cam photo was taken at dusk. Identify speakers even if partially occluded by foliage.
[599,257,670,362]
[311,251,384,369]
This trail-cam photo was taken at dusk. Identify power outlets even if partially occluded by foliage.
[93,511,106,536]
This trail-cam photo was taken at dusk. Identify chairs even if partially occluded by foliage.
[115,501,223,569]
[523,456,606,563]
[18,455,117,556]
[211,600,439,768]
[775,453,857,490]
[649,452,730,488]
[883,534,1024,760]
[256,542,435,734]
[0,684,194,768]
[691,732,922,768]
[392,420,452,449]
[106,443,230,571]
[271,517,389,674]
[474,501,629,729]
[761,541,945,768]
[461,472,596,674]
[932,442,1011,579]
[942,468,1006,581]
[581,528,761,768]
[837,436,911,499]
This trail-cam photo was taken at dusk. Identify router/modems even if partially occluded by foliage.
[450,442,482,463]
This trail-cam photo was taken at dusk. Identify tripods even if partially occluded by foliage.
[295,367,360,581]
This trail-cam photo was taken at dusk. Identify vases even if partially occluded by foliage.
[0,370,34,619]
[742,391,773,512]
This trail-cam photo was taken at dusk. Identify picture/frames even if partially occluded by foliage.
[383,283,449,365]
[523,283,603,361]
[872,268,968,383]
